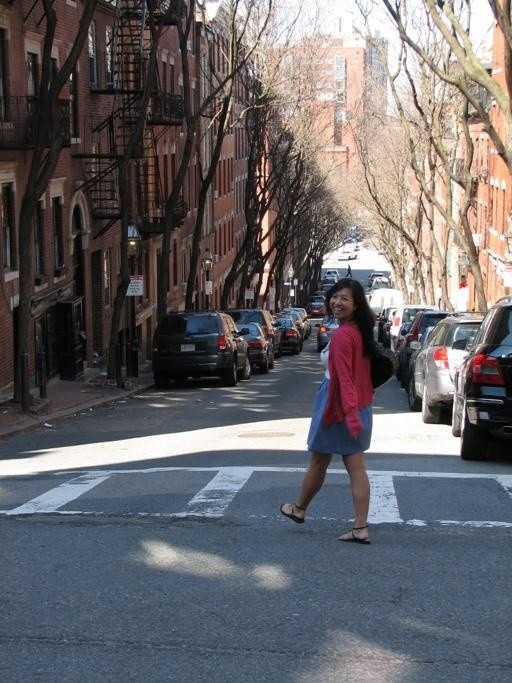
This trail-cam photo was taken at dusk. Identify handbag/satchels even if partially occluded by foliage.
[371,344,393,388]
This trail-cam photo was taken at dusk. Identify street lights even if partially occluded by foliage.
[121,220,145,378]
[201,247,217,312]
[244,261,251,308]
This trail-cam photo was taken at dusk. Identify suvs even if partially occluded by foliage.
[406,314,485,423]
[149,309,255,389]
[450,293,512,462]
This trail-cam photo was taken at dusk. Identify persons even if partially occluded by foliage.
[345,264,352,278]
[279,276,376,545]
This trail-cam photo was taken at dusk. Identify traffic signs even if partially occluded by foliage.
[126,272,144,297]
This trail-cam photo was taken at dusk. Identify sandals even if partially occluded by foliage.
[281,503,305,524]
[337,526,371,545]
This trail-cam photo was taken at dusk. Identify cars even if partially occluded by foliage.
[336,225,365,262]
[225,271,470,392]
